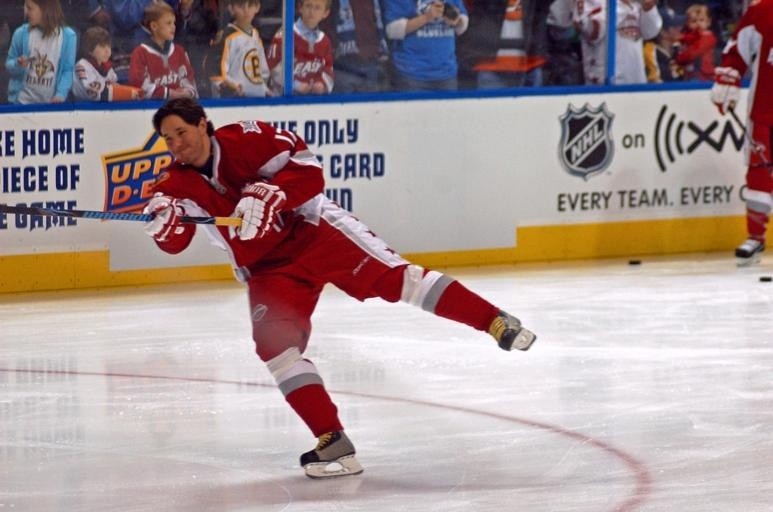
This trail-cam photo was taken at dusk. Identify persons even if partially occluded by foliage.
[143,97,521,466]
[710,0,773,258]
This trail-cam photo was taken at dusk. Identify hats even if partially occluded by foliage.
[655,3,690,28]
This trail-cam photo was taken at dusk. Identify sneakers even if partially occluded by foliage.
[484,305,524,354]
[297,425,358,469]
[733,234,768,260]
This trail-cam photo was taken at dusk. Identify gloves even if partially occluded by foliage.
[223,178,291,249]
[707,64,744,118]
[137,191,192,246]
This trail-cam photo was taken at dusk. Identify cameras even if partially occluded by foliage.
[444,3,457,20]
